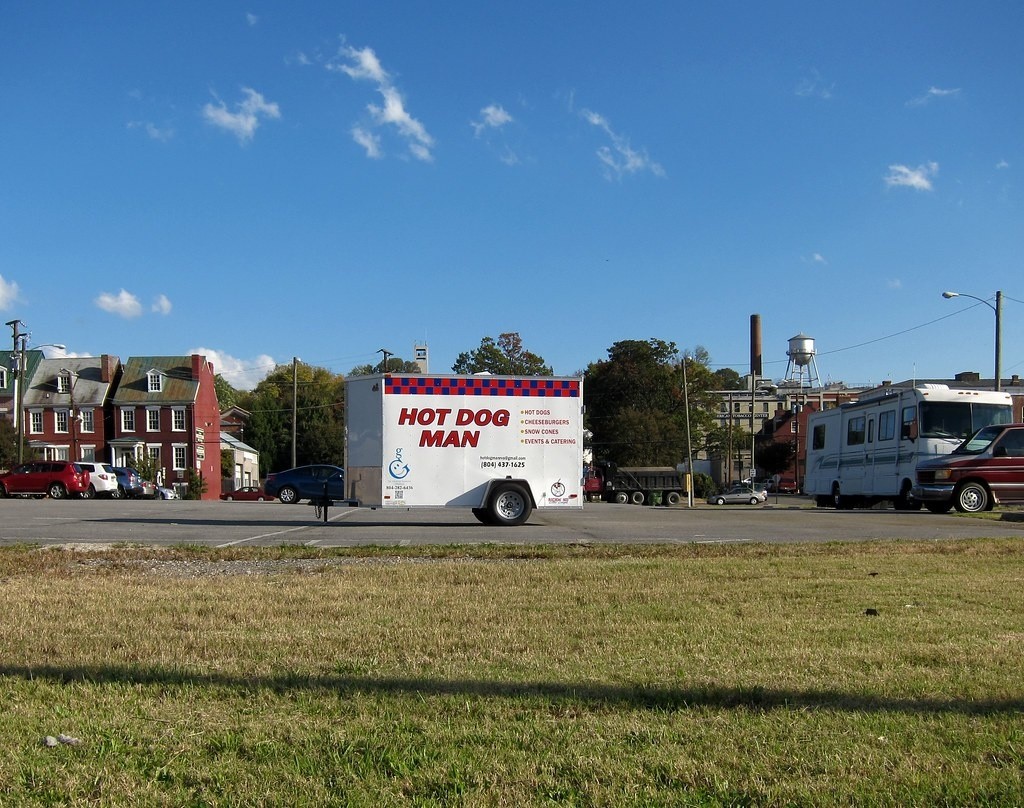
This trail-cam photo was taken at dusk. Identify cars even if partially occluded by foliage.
[740,477,797,501]
[707,487,765,505]
[263,464,358,506]
[219,486,276,501]
[126,476,176,500]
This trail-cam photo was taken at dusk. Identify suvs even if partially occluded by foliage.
[910,423,1024,513]
[0,460,91,500]
[112,466,144,499]
[74,461,118,500]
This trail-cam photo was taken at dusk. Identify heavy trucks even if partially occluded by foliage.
[584,462,684,506]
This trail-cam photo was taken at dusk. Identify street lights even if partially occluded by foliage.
[942,289,1004,392]
[751,369,778,491]
[290,357,302,468]
[18,338,66,466]
[680,356,695,506]
[727,392,756,487]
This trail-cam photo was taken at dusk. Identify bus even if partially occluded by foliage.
[801,382,1014,511]
